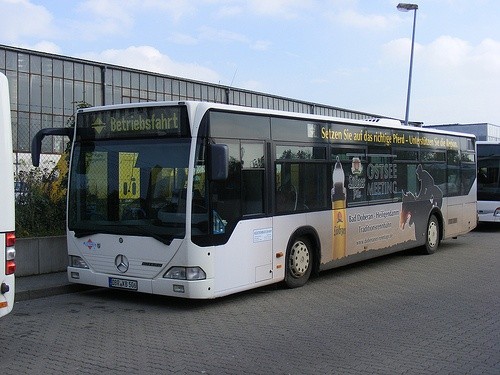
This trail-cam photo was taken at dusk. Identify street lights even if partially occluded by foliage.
[396,2,418,125]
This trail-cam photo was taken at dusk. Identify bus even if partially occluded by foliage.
[31,100,479,300]
[477,141,500,223]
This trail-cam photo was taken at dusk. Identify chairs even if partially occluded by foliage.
[277,183,297,211]
